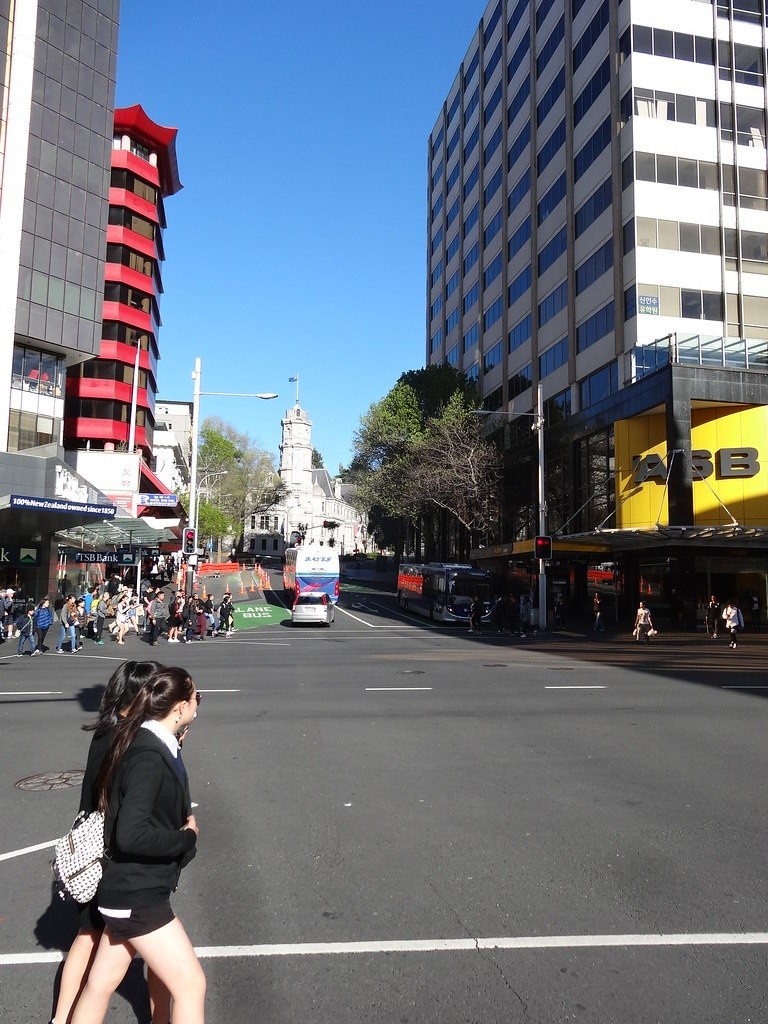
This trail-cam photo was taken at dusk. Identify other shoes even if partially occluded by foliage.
[729,642,737,648]
[78,647,83,650]
[167,638,180,643]
[71,649,78,653]
[94,640,104,645]
[211,630,216,639]
[183,635,187,641]
[225,635,233,638]
[200,637,206,641]
[56,649,65,654]
[712,633,720,640]
[151,641,160,647]
[30,649,40,657]
[186,640,192,644]
[17,653,23,658]
[40,652,44,655]
[496,631,504,635]
[467,629,474,633]
[521,633,527,638]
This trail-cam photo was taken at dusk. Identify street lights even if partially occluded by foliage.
[195,470,228,529]
[185,357,278,604]
[469,384,547,631]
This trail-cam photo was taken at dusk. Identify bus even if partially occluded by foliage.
[396,562,504,623]
[280,545,340,605]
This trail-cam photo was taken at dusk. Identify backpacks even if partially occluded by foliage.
[50,808,107,904]
[217,604,224,619]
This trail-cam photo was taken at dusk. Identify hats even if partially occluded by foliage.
[7,588,15,595]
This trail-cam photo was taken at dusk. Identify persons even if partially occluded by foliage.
[553,592,568,631]
[707,588,762,648]
[0,555,239,657]
[634,601,653,643]
[467,591,537,637]
[592,591,608,632]
[47,659,207,1024]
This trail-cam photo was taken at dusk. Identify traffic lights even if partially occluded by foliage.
[534,536,552,560]
[182,528,196,554]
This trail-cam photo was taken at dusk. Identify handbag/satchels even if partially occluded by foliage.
[632,627,638,637]
[14,629,22,639]
[647,628,658,636]
[68,616,76,627]
[736,623,744,633]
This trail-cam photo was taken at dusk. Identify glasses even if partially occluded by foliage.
[174,692,202,711]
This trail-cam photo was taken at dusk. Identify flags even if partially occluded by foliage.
[289,377,297,382]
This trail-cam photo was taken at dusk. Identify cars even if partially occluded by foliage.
[291,592,335,627]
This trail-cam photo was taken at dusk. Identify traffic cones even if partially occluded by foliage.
[250,561,271,592]
[201,584,207,598]
[240,583,244,594]
[241,563,246,571]
[224,583,230,593]
